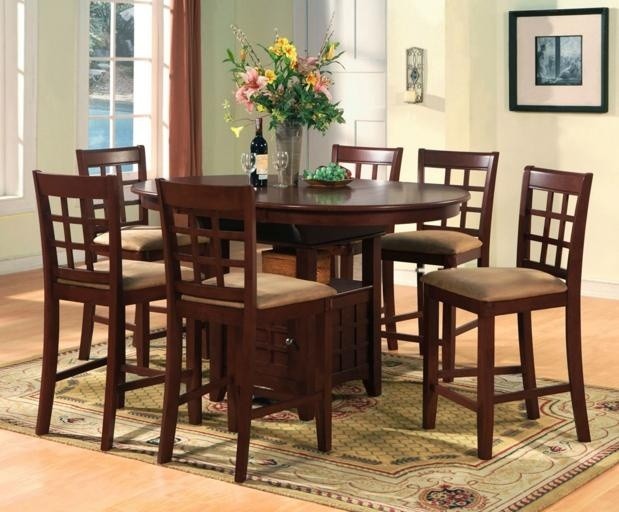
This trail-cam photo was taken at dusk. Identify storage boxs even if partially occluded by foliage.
[260,247,333,290]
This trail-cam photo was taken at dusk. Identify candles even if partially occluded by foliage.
[402,87,417,102]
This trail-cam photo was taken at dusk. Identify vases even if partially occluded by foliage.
[270,115,304,189]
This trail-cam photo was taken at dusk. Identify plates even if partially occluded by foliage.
[300,177,353,189]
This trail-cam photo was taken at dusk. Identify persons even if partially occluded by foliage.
[537,44,545,80]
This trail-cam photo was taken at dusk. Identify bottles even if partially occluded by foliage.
[250,117,269,188]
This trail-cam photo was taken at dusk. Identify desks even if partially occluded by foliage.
[126,172,474,423]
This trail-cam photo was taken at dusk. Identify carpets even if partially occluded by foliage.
[0,323,619,511]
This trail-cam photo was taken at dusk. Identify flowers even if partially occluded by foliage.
[215,9,350,139]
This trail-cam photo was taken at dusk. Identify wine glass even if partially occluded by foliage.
[272,151,289,187]
[241,152,258,191]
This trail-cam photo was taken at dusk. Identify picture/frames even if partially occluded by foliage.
[504,6,611,116]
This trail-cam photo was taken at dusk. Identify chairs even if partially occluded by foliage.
[313,141,407,354]
[71,144,216,376]
[25,166,209,453]
[149,173,343,485]
[378,145,504,383]
[414,162,600,463]
